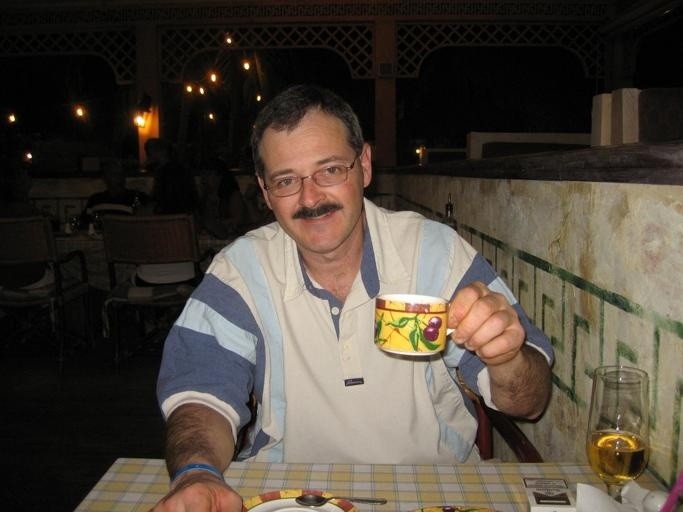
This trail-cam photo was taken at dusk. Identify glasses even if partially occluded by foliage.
[262,152,359,197]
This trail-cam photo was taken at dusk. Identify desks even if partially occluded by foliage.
[50,227,240,281]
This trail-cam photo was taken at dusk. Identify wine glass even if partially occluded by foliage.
[584,365,653,506]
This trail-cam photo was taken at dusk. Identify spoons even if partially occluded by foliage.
[294,492,388,506]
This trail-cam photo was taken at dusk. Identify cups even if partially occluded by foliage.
[372,291,458,357]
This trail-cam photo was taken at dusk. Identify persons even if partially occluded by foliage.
[0,143,270,292]
[154,84,556,512]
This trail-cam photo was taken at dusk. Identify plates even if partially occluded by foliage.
[239,487,359,512]
[409,505,502,512]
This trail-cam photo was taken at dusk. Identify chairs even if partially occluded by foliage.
[0,214,93,391]
[83,205,136,222]
[232,372,546,463]
[103,214,203,376]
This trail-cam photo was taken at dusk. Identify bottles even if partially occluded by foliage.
[62,212,106,241]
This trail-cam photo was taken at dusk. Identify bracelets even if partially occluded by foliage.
[173,464,225,481]
